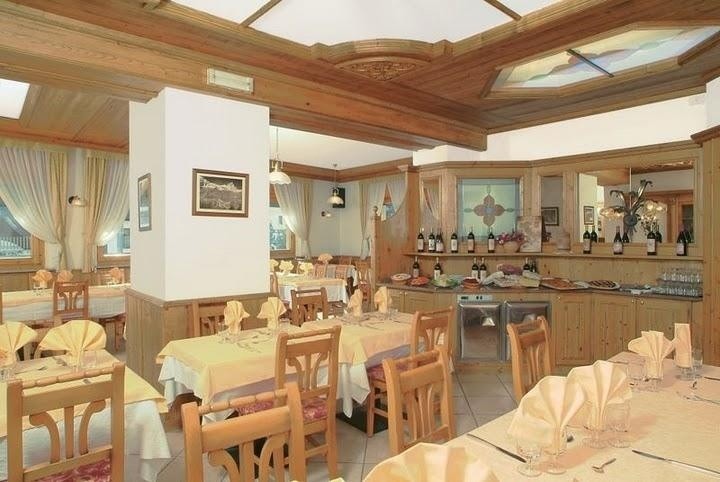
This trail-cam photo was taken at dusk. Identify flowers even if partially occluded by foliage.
[494,231,528,246]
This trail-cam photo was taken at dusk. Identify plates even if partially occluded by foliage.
[539,279,621,290]
[410,277,429,285]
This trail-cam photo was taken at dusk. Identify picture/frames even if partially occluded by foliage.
[191,167,250,220]
[138,172,152,232]
[583,206,595,225]
[541,206,559,226]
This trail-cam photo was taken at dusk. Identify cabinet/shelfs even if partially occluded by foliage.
[678,199,694,242]
[552,292,704,369]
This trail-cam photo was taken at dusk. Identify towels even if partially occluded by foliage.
[566,360,626,427]
[39,320,107,365]
[57,271,74,280]
[32,269,52,282]
[0,322,38,363]
[507,376,587,454]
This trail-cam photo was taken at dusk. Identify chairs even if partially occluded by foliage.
[7,361,124,482]
[95,268,129,352]
[53,278,90,322]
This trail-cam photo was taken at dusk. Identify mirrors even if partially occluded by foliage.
[575,156,697,247]
[539,176,563,242]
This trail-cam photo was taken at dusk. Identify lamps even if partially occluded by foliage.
[68,195,82,206]
[328,163,344,205]
[599,167,666,235]
[268,127,294,184]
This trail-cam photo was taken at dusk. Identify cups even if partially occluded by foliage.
[0,348,98,387]
[215,303,398,345]
[654,270,704,296]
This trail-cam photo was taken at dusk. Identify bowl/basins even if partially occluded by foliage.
[461,280,479,289]
[431,280,457,288]
[391,274,412,285]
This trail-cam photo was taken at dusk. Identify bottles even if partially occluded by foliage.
[582,226,592,254]
[613,226,623,254]
[655,225,663,243]
[417,227,496,255]
[647,225,657,256]
[471,256,487,283]
[676,232,687,256]
[591,226,598,243]
[412,256,421,277]
[685,231,691,242]
[521,256,537,277]
[621,229,629,243]
[433,257,441,280]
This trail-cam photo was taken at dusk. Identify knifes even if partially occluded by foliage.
[466,433,524,462]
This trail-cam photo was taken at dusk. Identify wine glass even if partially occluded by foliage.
[513,345,720,479]
[30,274,121,298]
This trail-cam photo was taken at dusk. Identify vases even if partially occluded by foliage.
[501,241,519,253]
